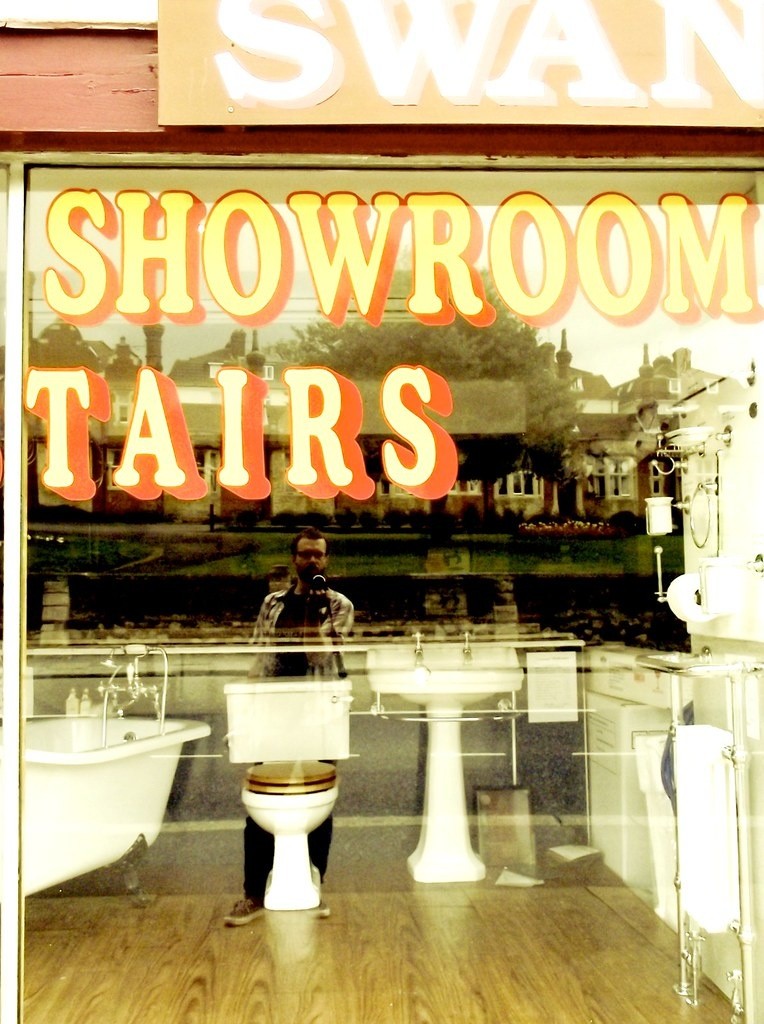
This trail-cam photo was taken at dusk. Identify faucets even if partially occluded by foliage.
[411,632,425,656]
[462,631,473,657]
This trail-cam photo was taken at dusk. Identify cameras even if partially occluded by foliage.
[309,574,327,592]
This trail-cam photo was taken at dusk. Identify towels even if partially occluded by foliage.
[672,723,741,936]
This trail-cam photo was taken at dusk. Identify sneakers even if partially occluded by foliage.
[224,898,266,926]
[317,900,330,918]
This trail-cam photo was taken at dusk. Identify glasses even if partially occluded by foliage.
[296,550,327,559]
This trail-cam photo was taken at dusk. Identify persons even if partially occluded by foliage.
[223,525,356,928]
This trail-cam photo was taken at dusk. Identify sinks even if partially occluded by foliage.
[366,658,526,697]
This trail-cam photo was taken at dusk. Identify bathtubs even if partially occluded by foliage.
[20,710,216,904]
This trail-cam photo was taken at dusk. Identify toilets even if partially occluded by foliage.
[224,679,356,910]
